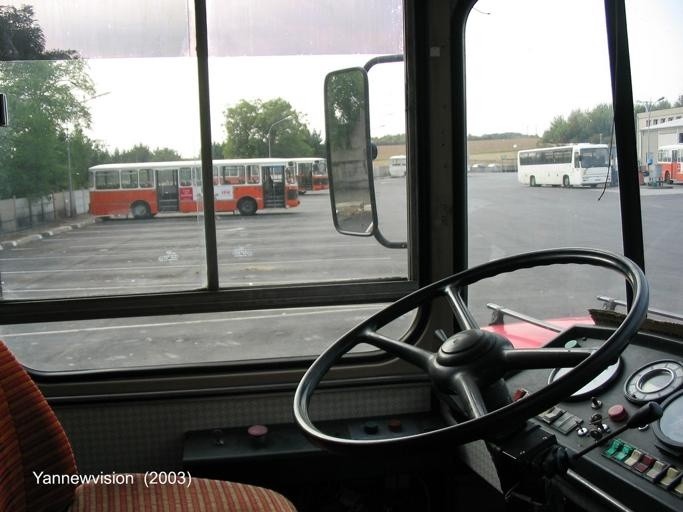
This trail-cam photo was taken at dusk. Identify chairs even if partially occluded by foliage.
[0,340,297,512]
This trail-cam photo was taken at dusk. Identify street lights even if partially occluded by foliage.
[65,90,112,218]
[636,95,664,175]
[267,115,295,158]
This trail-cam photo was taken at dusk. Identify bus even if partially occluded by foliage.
[294,157,328,196]
[516,142,611,188]
[388,154,406,178]
[655,143,683,184]
[86,157,301,220]
